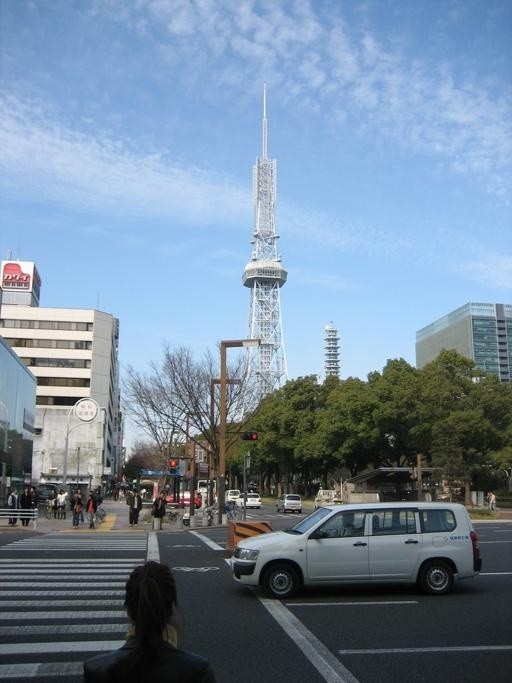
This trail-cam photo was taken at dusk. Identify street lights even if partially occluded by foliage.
[208,375,242,523]
[169,429,180,457]
[184,407,211,490]
[73,445,80,491]
[218,337,263,525]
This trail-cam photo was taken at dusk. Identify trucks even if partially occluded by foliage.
[314,490,344,510]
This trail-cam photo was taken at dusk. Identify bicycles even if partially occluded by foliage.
[165,499,244,526]
[45,505,62,520]
[86,506,106,522]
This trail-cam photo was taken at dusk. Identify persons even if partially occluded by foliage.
[8,481,202,530]
[336,513,358,537]
[448,485,465,503]
[83,560,217,682]
[486,492,497,512]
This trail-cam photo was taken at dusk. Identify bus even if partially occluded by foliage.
[34,482,71,505]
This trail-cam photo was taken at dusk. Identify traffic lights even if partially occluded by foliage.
[241,432,259,441]
[168,459,178,474]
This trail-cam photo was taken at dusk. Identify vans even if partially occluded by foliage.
[275,493,303,514]
[231,499,484,602]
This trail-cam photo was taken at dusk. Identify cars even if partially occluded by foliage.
[164,479,263,508]
